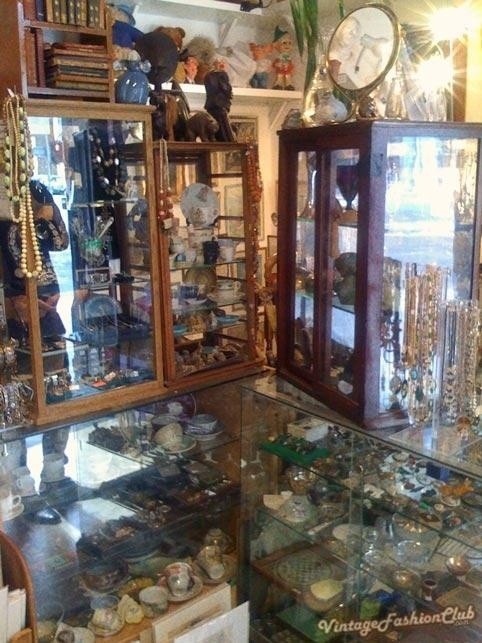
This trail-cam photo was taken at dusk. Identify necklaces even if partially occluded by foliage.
[0,92,45,279]
[383,256,482,439]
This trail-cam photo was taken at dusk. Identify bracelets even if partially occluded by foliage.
[0,346,29,423]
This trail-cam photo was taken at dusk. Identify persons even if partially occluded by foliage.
[0,180,104,524]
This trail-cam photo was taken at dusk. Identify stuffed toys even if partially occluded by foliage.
[107,3,296,90]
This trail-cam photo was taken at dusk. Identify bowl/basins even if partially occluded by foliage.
[189,415,219,433]
[89,595,119,612]
[218,246,234,261]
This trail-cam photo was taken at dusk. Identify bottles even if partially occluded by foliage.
[114,58,149,105]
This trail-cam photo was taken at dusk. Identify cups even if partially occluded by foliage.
[180,284,199,300]
[138,586,169,619]
[0,493,22,515]
[202,240,220,267]
[444,556,472,591]
[196,546,224,578]
[164,561,190,597]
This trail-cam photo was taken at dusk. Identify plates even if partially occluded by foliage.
[87,609,127,638]
[154,435,197,454]
[183,426,226,442]
[185,264,220,301]
[0,502,24,521]
[181,184,220,227]
[333,523,347,541]
[183,553,239,585]
[154,574,204,605]
[54,628,93,643]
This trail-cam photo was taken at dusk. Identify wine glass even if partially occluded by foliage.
[358,525,382,577]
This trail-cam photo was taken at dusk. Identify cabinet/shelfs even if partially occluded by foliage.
[1,0,482,643]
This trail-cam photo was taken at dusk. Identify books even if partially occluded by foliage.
[21,0,108,91]
